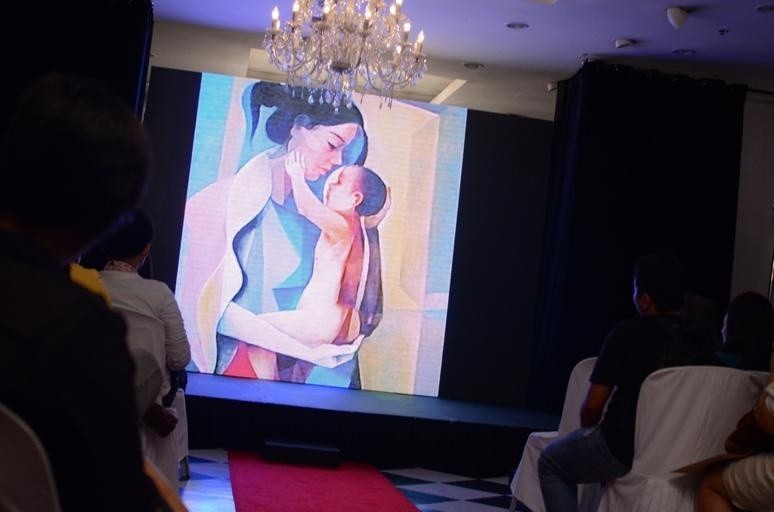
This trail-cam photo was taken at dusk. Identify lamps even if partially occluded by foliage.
[262,1,431,113]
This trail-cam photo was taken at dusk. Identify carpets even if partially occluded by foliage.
[220,443,419,512]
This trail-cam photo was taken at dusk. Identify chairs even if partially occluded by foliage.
[512,356,770,512]
